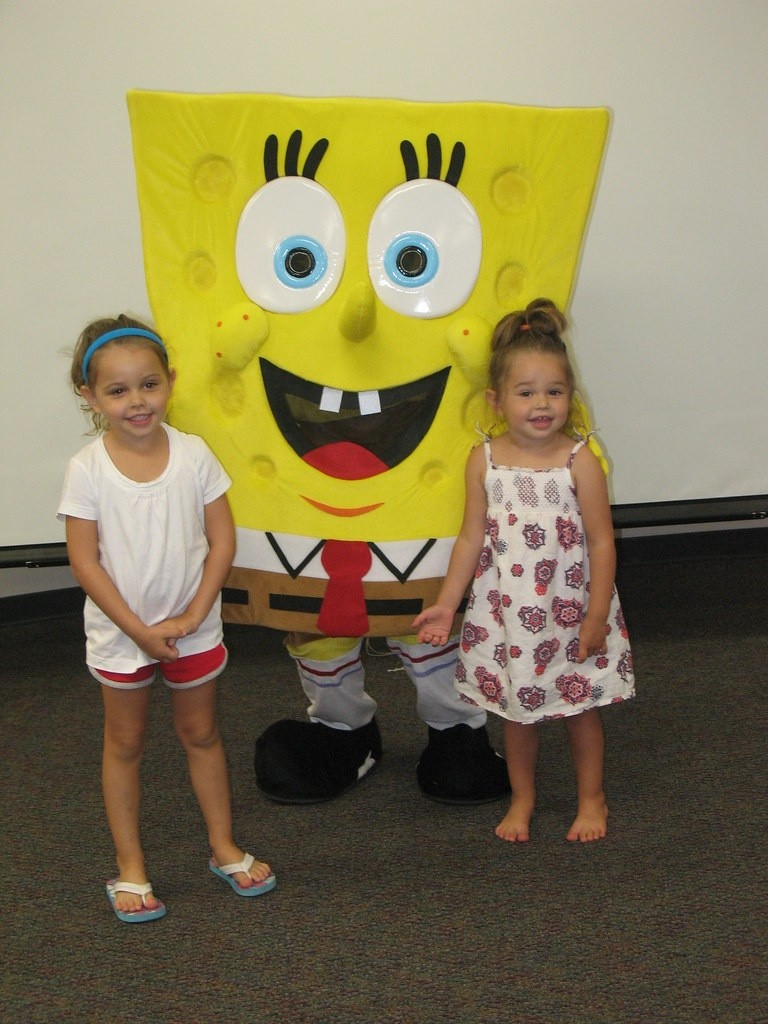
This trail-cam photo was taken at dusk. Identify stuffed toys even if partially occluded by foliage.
[120,89,609,802]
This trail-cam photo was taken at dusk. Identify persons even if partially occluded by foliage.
[412,298,638,841]
[56,314,275,922]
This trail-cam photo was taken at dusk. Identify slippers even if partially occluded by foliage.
[106,878,166,923]
[209,852,276,896]
